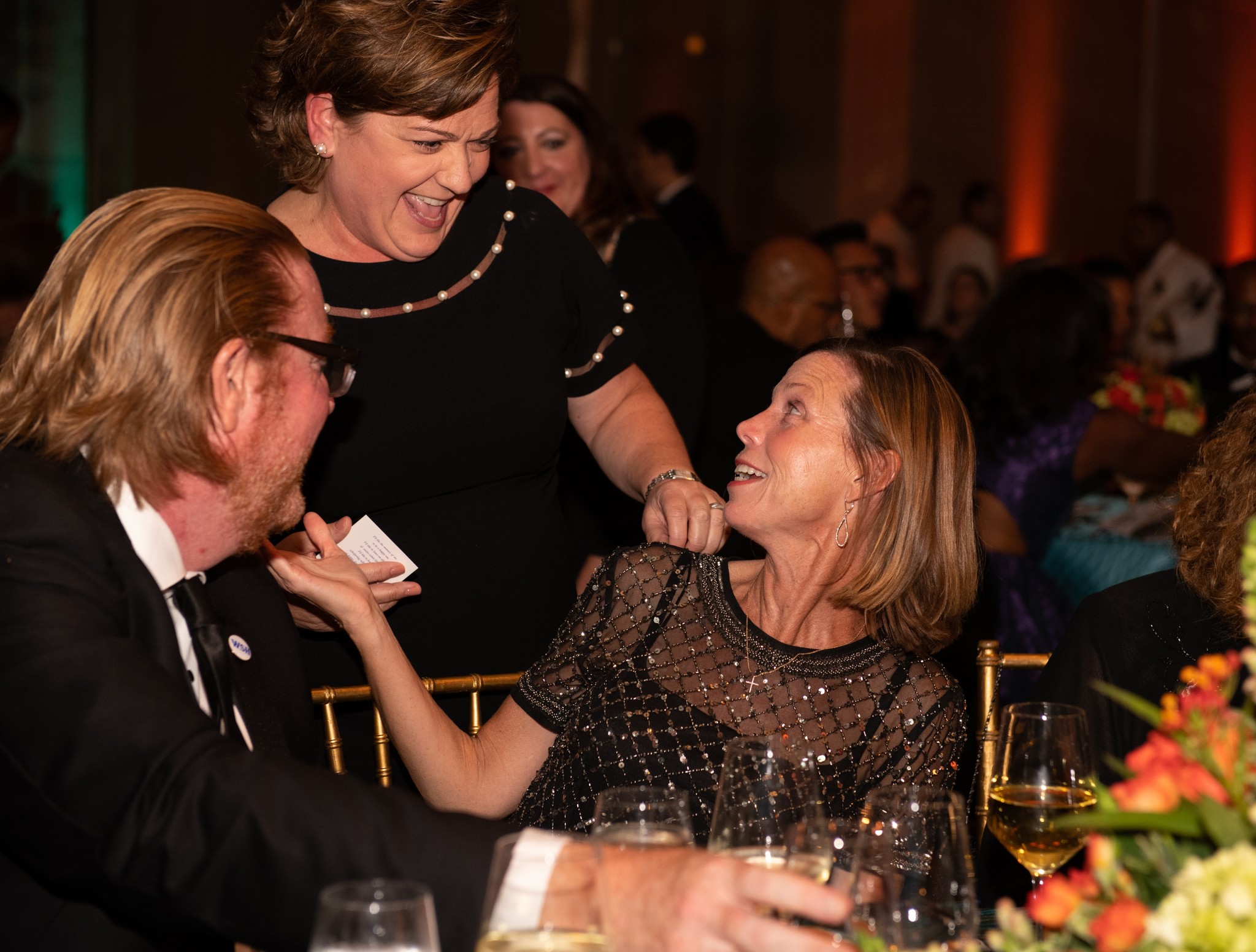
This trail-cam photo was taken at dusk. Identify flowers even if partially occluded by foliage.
[948,515,1256,952]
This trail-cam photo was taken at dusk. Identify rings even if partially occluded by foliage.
[709,502,724,511]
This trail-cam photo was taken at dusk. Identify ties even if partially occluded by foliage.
[175,575,238,739]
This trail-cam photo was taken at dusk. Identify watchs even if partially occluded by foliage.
[645,469,703,498]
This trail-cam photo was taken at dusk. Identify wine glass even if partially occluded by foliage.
[986,703,1097,941]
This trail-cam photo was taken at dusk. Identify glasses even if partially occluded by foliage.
[246,330,359,397]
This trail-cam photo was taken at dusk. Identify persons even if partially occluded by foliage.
[633,110,1256,915]
[0,186,861,952]
[490,74,766,652]
[268,339,980,952]
[246,0,730,798]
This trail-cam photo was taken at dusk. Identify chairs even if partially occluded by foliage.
[308,674,539,784]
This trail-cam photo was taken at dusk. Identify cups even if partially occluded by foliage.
[590,737,979,952]
[308,877,441,950]
